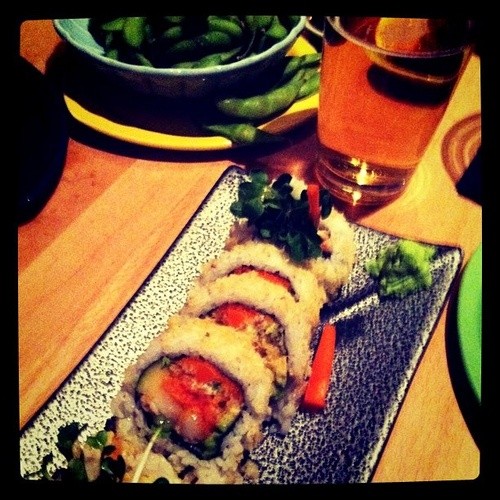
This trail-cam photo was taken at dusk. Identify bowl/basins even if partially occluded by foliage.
[52,15,306,113]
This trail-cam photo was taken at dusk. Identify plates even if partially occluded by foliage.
[18,55,70,226]
[20,166,462,483]
[62,35,322,152]
[458,239,482,407]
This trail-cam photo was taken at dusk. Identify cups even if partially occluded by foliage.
[315,14,477,205]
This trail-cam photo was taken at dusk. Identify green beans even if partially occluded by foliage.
[89,13,322,146]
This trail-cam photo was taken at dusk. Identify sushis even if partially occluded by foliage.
[109,174,360,486]
